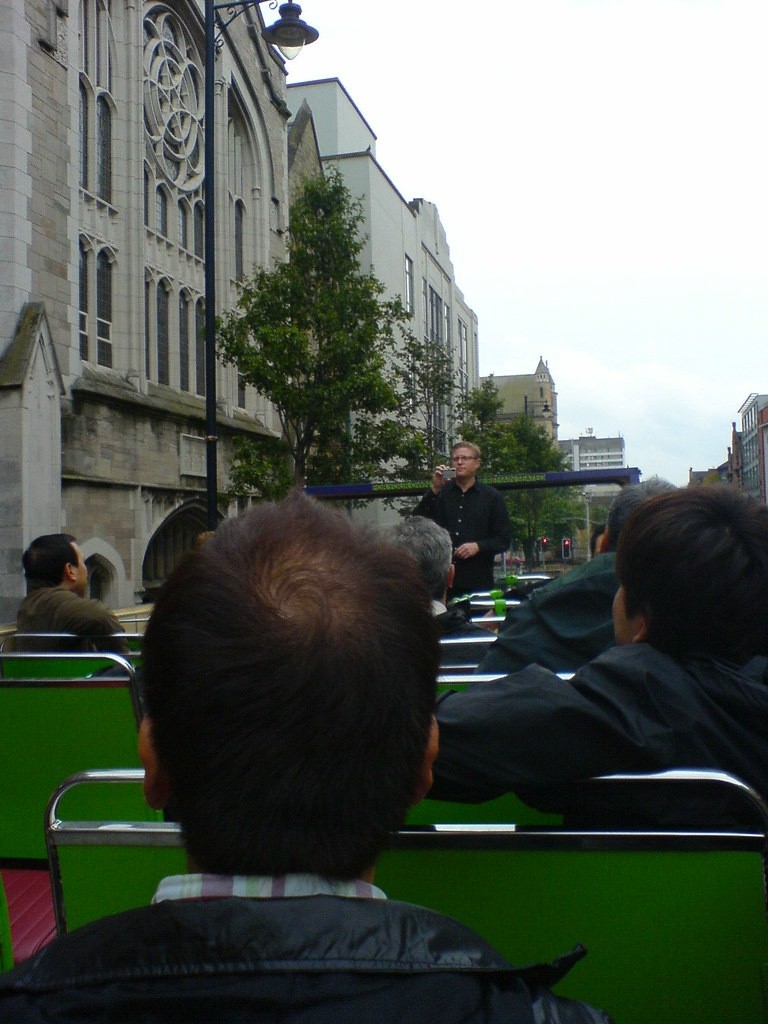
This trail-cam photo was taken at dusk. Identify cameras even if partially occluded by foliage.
[441,468,457,481]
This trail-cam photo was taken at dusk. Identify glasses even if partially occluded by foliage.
[452,456,476,461]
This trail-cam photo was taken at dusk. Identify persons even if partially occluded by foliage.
[427,486,768,834]
[413,442,511,603]
[473,481,677,675]
[386,516,499,676]
[16,533,131,663]
[0,498,607,1024]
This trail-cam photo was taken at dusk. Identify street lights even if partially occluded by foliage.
[204,0,319,530]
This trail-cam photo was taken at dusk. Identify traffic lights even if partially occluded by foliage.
[562,538,571,559]
[541,538,549,552]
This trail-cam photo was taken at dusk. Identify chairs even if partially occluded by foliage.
[0,571,768,1022]
